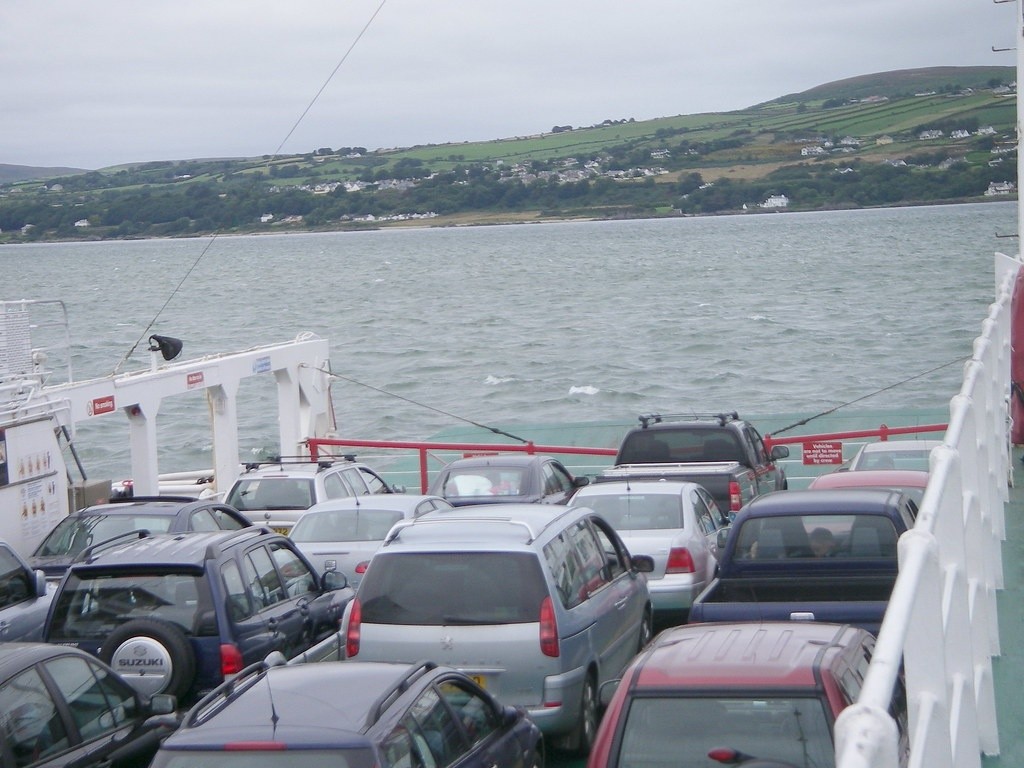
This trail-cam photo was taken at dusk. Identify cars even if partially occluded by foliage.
[0,538,65,651]
[564,481,730,621]
[0,638,185,768]
[798,468,935,543]
[582,611,911,767]
[420,453,591,517]
[280,493,457,594]
[836,439,945,476]
[24,490,255,597]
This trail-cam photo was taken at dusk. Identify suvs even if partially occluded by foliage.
[141,659,550,768]
[215,452,391,549]
[44,526,354,723]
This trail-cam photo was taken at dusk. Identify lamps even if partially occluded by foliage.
[148,334,183,361]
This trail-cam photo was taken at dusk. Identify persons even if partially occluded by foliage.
[810,527,834,555]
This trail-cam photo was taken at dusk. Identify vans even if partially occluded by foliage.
[341,500,656,760]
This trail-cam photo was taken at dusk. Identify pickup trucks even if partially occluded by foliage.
[678,483,921,681]
[582,411,790,526]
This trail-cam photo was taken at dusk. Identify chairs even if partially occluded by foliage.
[645,441,670,460]
[757,516,817,558]
[871,460,894,470]
[175,580,198,609]
[704,440,725,459]
[849,516,898,557]
[646,699,727,734]
